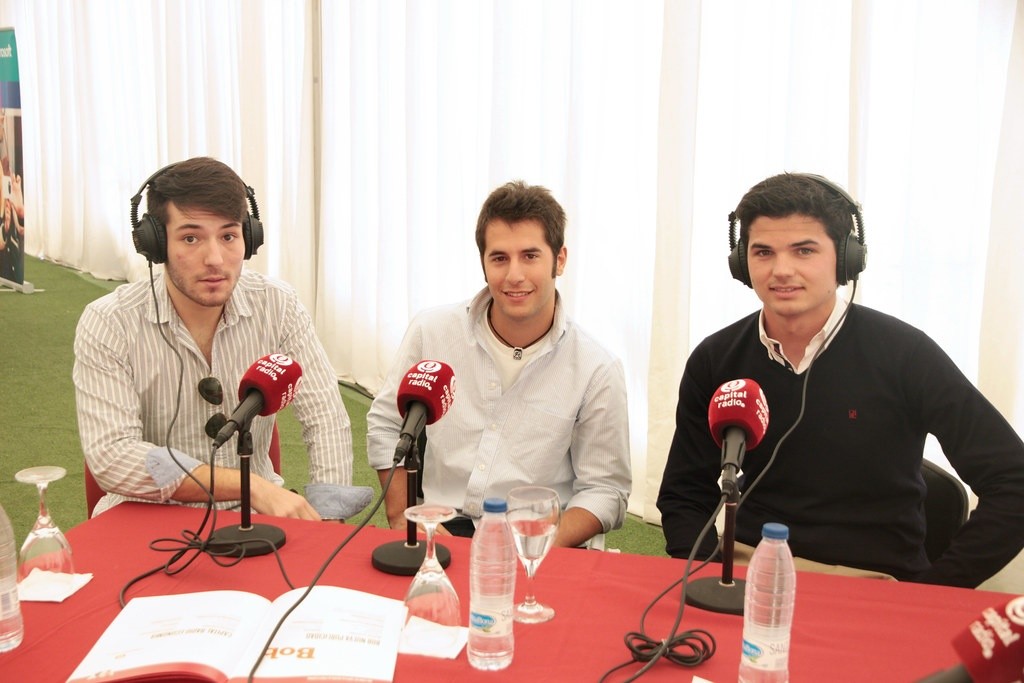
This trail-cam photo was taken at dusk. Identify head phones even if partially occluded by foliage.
[727,174,867,287]
[130,162,264,264]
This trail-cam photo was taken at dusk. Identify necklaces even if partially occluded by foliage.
[488,300,555,360]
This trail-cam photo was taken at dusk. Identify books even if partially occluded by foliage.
[66,584,409,683]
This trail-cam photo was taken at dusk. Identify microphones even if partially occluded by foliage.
[707,379,769,496]
[213,354,303,449]
[916,593,1024,683]
[393,360,456,462]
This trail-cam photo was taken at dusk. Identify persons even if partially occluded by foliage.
[72,157,375,524]
[656,173,1024,593]
[367,179,632,550]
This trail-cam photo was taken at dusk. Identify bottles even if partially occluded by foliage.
[0,504,24,653]
[468,498,516,671]
[739,523,796,683]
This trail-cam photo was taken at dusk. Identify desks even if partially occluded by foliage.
[1,498,1020,683]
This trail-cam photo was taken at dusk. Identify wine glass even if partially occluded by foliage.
[506,486,561,625]
[404,504,461,643]
[15,466,76,599]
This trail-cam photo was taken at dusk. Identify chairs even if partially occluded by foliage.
[920,457,969,563]
[84,419,282,519]
